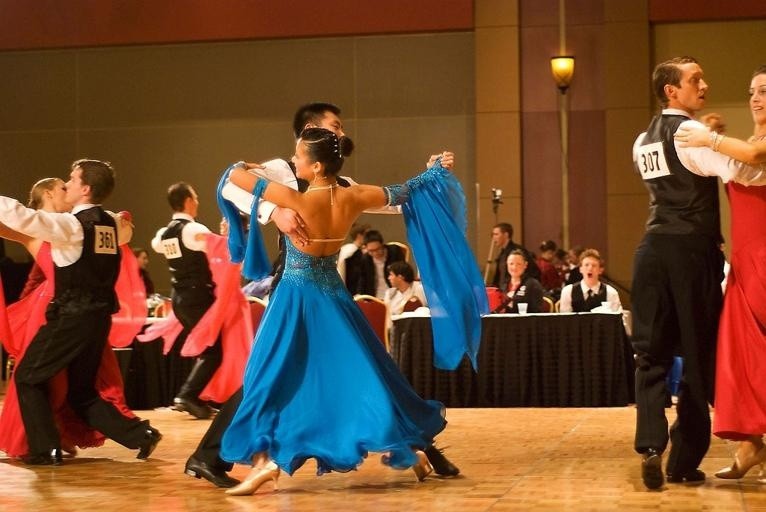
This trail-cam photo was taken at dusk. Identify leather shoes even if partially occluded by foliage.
[665,468,706,485]
[641,448,664,489]
[183,454,242,489]
[423,445,461,477]
[172,396,213,420]
[136,427,162,459]
[21,447,65,468]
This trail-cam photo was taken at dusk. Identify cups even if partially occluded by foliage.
[516,302,529,313]
[602,301,613,312]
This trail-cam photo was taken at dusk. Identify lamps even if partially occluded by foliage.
[550,55,575,95]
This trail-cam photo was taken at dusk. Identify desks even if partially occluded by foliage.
[389,311,634,407]
[112,316,200,410]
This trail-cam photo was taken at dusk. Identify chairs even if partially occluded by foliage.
[154,293,389,354]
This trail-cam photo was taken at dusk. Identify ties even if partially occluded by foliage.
[588,288,592,300]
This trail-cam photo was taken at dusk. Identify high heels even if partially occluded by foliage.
[225,460,281,496]
[713,443,766,482]
[412,450,433,482]
